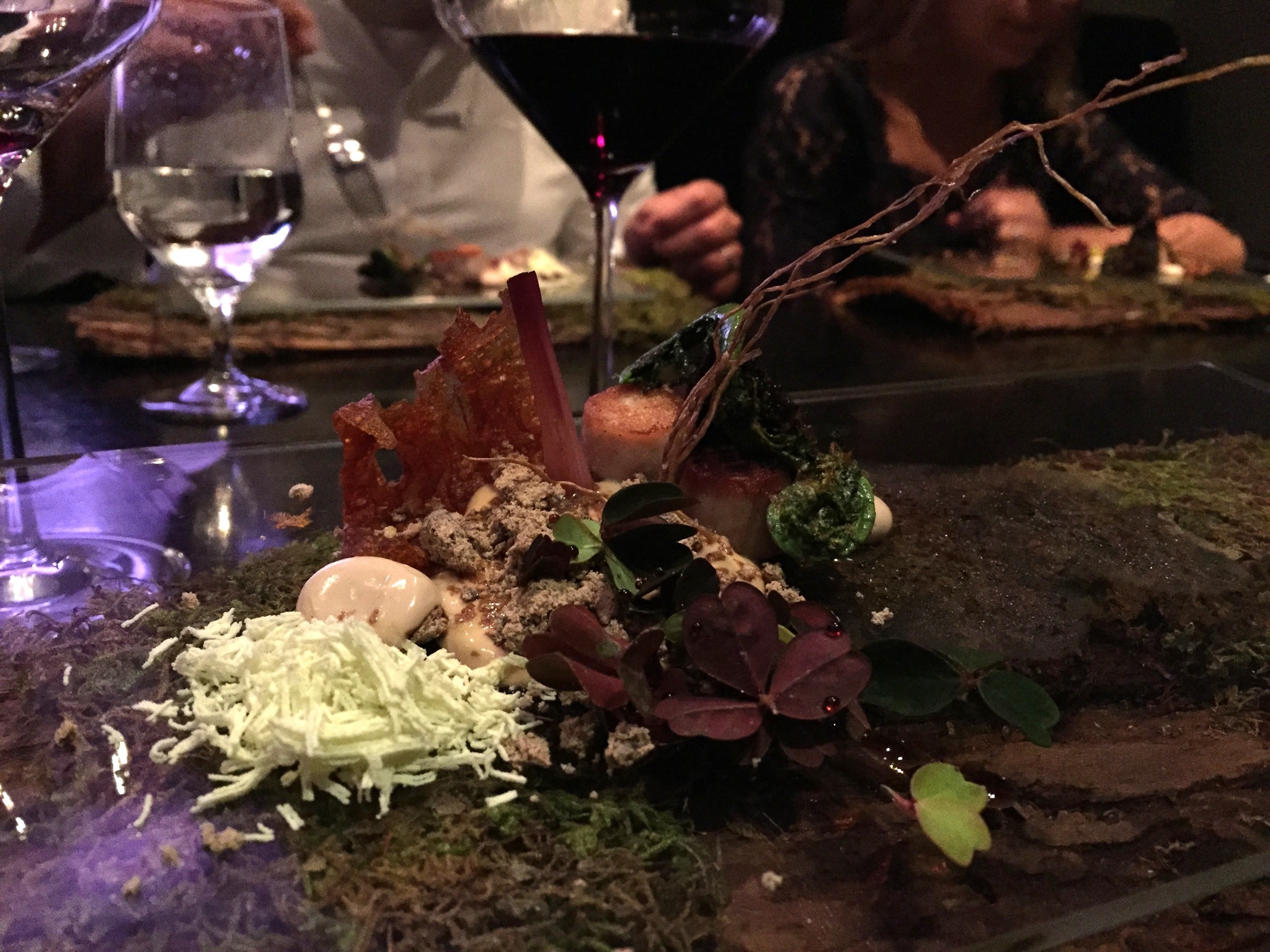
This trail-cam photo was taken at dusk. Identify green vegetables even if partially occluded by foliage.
[510,300,1063,864]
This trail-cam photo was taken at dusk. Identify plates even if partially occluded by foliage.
[161,245,596,312]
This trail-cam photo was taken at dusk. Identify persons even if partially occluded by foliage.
[0,0,745,302]
[739,0,1270,341]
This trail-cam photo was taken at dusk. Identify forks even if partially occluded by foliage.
[290,56,386,225]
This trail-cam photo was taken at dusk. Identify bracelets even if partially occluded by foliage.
[964,188,981,205]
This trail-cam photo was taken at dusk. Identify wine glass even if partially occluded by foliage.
[106,0,311,425]
[433,0,780,389]
[0,0,193,653]
